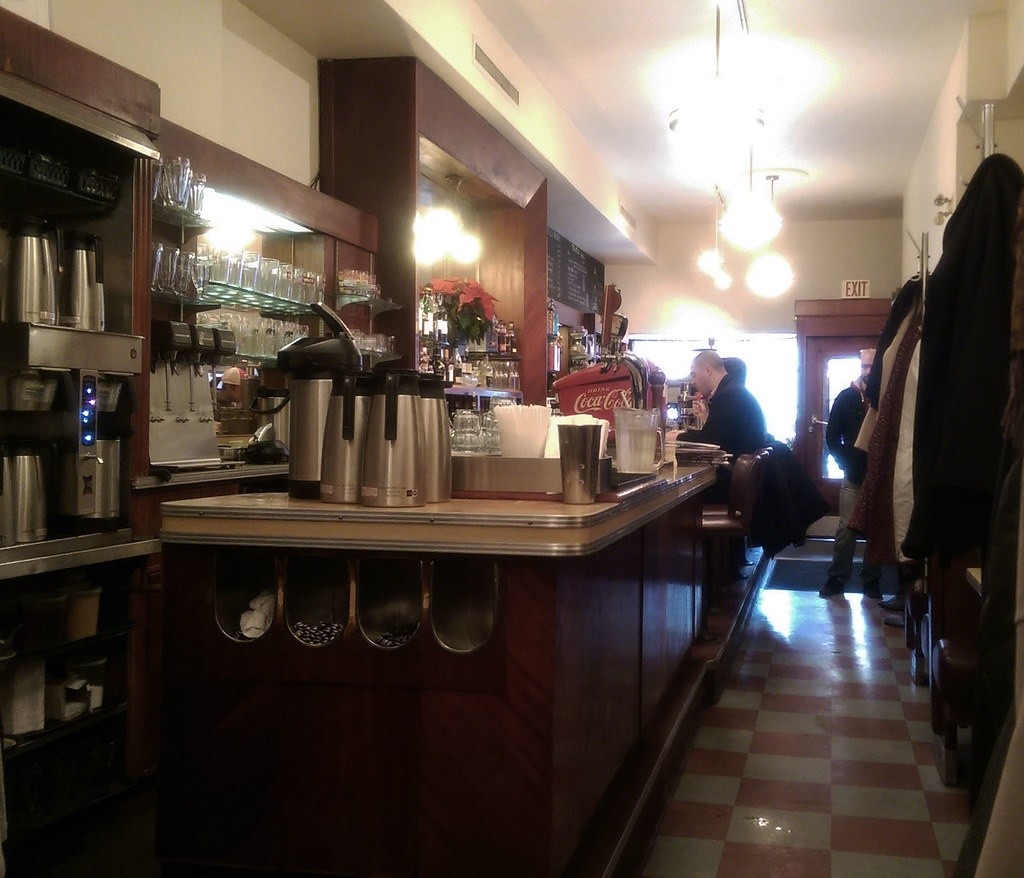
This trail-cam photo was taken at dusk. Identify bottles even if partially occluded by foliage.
[420,329,462,387]
[498,320,517,353]
[481,354,493,388]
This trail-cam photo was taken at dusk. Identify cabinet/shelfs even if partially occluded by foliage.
[0,73,153,877]
[154,116,376,469]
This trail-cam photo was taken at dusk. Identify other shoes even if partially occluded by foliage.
[819,578,844,598]
[884,617,905,627]
[863,580,883,598]
[878,596,905,610]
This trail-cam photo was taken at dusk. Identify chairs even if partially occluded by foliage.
[703,440,831,613]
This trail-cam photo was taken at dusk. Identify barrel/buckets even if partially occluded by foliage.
[0,585,103,650]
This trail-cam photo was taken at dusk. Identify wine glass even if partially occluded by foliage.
[191,264,207,300]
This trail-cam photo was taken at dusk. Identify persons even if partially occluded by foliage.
[818,348,887,601]
[692,355,747,422]
[663,348,768,505]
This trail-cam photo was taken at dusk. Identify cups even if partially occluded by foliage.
[473,361,522,390]
[152,241,319,299]
[249,388,288,444]
[150,157,206,215]
[202,314,310,357]
[452,410,499,454]
[351,329,395,354]
[558,424,602,504]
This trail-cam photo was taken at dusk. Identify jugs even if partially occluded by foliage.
[419,380,454,503]
[63,231,105,331]
[1,217,60,324]
[289,371,343,497]
[0,443,45,546]
[321,373,374,502]
[362,372,424,507]
[614,407,665,472]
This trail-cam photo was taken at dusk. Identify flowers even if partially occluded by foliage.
[422,277,499,343]
